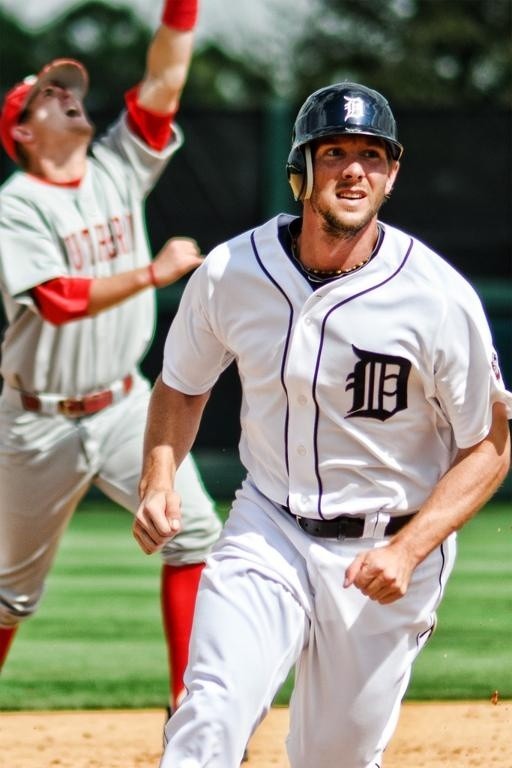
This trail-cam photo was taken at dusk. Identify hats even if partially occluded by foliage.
[0,60,85,159]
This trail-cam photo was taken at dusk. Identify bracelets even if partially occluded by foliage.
[159,1,199,33]
[148,263,160,288]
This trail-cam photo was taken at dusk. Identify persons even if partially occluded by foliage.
[0,1,248,764]
[132,83,512,767]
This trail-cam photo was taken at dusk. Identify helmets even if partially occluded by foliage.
[288,81,402,170]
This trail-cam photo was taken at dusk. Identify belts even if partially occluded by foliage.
[21,375,132,419]
[285,507,419,538]
